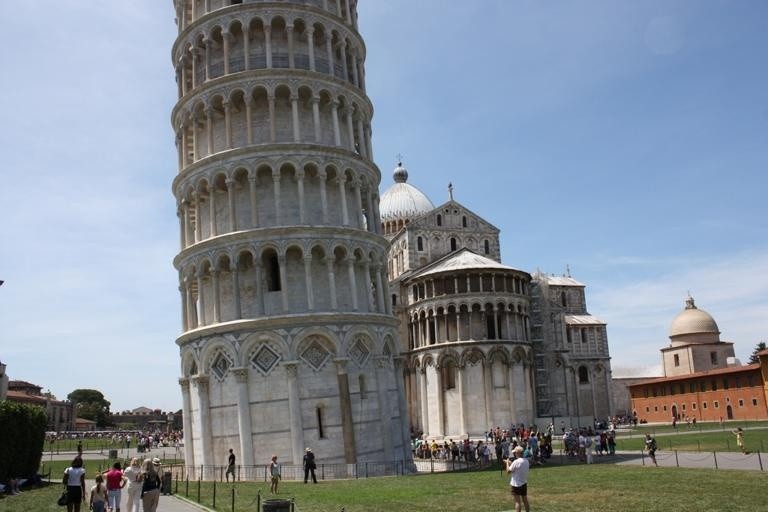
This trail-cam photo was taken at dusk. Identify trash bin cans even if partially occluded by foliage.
[262,499,290,512]
[164,472,171,495]
[109,450,117,458]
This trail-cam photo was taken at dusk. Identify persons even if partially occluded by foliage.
[502,445,530,512]
[224,447,235,485]
[644,432,660,467]
[691,414,697,425]
[0,478,25,495]
[672,414,676,428]
[40,428,185,511]
[5,474,48,487]
[685,415,692,425]
[269,454,282,496]
[302,447,318,484]
[732,425,750,455]
[410,413,638,469]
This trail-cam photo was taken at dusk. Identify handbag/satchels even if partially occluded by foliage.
[536,448,539,452]
[103,497,108,509]
[58,492,68,506]
[312,462,317,469]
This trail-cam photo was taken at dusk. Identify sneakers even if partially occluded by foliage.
[271,491,277,494]
[596,453,615,456]
[564,449,579,457]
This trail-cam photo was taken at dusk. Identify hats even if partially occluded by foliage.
[152,458,162,465]
[512,445,523,453]
[306,448,312,453]
